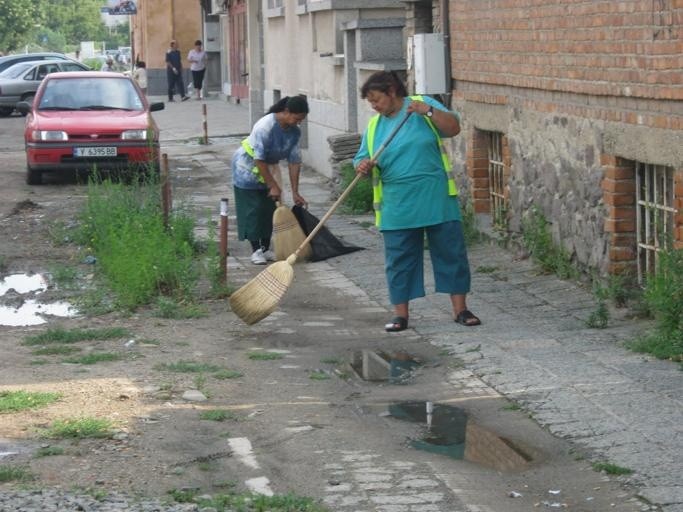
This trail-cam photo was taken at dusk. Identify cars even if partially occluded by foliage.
[104,46,130,64]
[14,71,166,186]
[1,50,94,117]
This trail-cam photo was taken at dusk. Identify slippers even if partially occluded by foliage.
[384,317,408,331]
[455,310,480,326]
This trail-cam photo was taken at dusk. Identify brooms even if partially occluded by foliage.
[269,198,313,265]
[226,113,415,328]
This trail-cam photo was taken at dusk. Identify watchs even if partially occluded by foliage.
[426,106,434,118]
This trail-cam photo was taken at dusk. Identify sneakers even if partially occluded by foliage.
[264,250,278,261]
[251,246,268,265]
[182,96,201,101]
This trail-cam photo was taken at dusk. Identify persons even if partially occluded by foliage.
[230,95,308,264]
[352,69,481,331]
[73,40,209,103]
